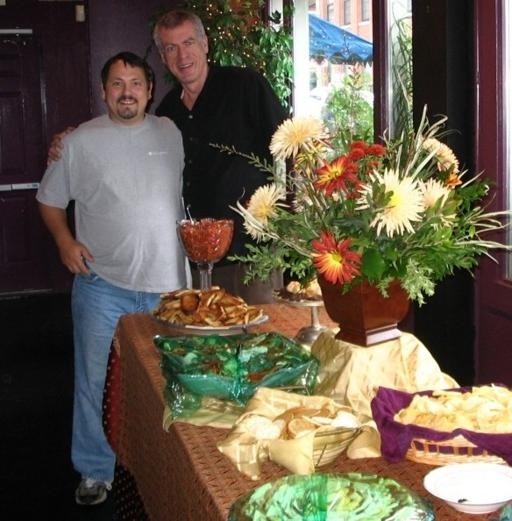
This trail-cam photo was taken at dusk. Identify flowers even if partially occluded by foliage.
[206,103,511,307]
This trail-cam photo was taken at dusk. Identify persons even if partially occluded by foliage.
[43,8,295,306]
[33,50,194,504]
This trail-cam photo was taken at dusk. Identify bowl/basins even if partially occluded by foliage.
[422,463,512,516]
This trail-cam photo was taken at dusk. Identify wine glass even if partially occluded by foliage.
[176,218,233,291]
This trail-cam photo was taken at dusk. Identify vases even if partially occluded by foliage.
[317,278,411,348]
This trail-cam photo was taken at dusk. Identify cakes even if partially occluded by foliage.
[233,470,427,521]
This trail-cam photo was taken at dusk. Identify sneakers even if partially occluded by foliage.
[76,477,112,505]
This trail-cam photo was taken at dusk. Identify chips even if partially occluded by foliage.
[393,382,512,434]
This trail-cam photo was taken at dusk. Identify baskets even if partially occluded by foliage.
[374,383,511,467]
[269,425,360,470]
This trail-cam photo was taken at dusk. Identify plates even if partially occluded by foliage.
[147,310,269,339]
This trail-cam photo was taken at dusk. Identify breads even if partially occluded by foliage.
[279,279,324,300]
[154,286,264,328]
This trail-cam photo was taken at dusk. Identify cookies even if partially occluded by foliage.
[237,402,362,442]
